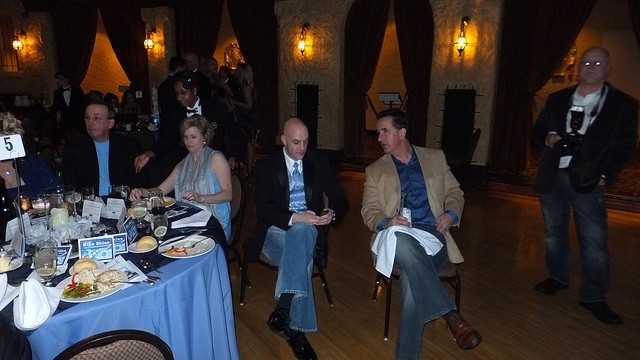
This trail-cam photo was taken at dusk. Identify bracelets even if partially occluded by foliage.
[329,208,337,224]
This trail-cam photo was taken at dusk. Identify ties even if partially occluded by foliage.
[292,163,300,181]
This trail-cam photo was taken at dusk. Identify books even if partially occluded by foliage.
[105,254,139,285]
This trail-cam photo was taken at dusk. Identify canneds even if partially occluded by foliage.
[400,208,411,228]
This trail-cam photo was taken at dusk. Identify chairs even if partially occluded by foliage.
[52,329,175,360]
[240,190,335,307]
[228,160,251,289]
[371,266,461,341]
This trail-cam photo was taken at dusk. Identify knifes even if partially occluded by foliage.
[160,231,202,248]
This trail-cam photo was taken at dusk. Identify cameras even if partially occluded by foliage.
[554,105,590,156]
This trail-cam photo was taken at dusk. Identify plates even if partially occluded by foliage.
[0,264,22,274]
[149,196,176,208]
[55,269,128,303]
[158,235,215,258]
[128,242,157,254]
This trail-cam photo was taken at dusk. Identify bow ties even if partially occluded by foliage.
[62,88,70,92]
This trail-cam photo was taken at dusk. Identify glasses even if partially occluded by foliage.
[82,115,111,122]
[583,62,601,68]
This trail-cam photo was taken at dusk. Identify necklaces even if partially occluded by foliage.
[1,168,14,176]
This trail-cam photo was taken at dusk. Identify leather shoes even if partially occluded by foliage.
[583,301,623,325]
[445,310,482,350]
[267,307,291,340]
[534,278,570,295]
[287,331,318,360]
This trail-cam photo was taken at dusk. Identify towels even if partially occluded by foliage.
[171,204,213,229]
[371,224,443,280]
[13,279,64,330]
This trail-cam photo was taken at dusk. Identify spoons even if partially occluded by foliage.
[138,259,163,276]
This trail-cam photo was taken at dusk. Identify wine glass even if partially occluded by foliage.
[32,194,47,217]
[152,197,165,217]
[116,185,130,203]
[82,186,95,203]
[131,200,147,228]
[34,243,57,288]
[65,189,81,215]
[151,215,168,245]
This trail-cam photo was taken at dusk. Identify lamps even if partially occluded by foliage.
[144,30,157,49]
[13,31,27,49]
[456,16,470,52]
[297,23,311,52]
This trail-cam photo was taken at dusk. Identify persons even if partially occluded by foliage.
[44,72,85,128]
[533,47,640,324]
[0,111,57,206]
[200,57,227,103]
[158,57,187,140]
[121,90,141,114]
[229,63,254,137]
[134,71,248,179]
[219,65,230,82]
[60,102,142,197]
[104,93,119,108]
[184,52,211,101]
[129,117,233,241]
[254,119,349,360]
[360,109,483,360]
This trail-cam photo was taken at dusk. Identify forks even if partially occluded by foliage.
[183,234,214,249]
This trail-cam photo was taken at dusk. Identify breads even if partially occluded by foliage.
[137,235,157,249]
[74,259,98,273]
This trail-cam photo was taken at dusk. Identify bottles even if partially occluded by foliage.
[53,157,65,193]
[399,192,413,227]
